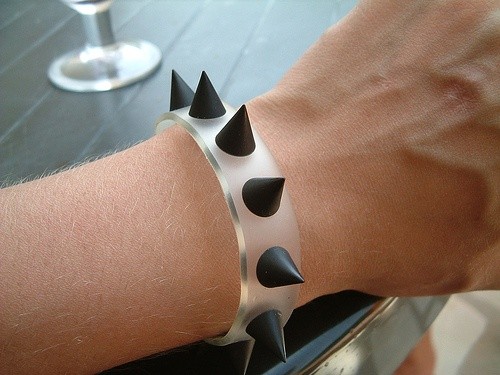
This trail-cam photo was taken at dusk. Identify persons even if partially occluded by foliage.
[1,0,500,374]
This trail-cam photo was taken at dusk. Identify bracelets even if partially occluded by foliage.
[154,67,305,375]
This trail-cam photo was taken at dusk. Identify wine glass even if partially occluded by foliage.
[46,0,162,93]
